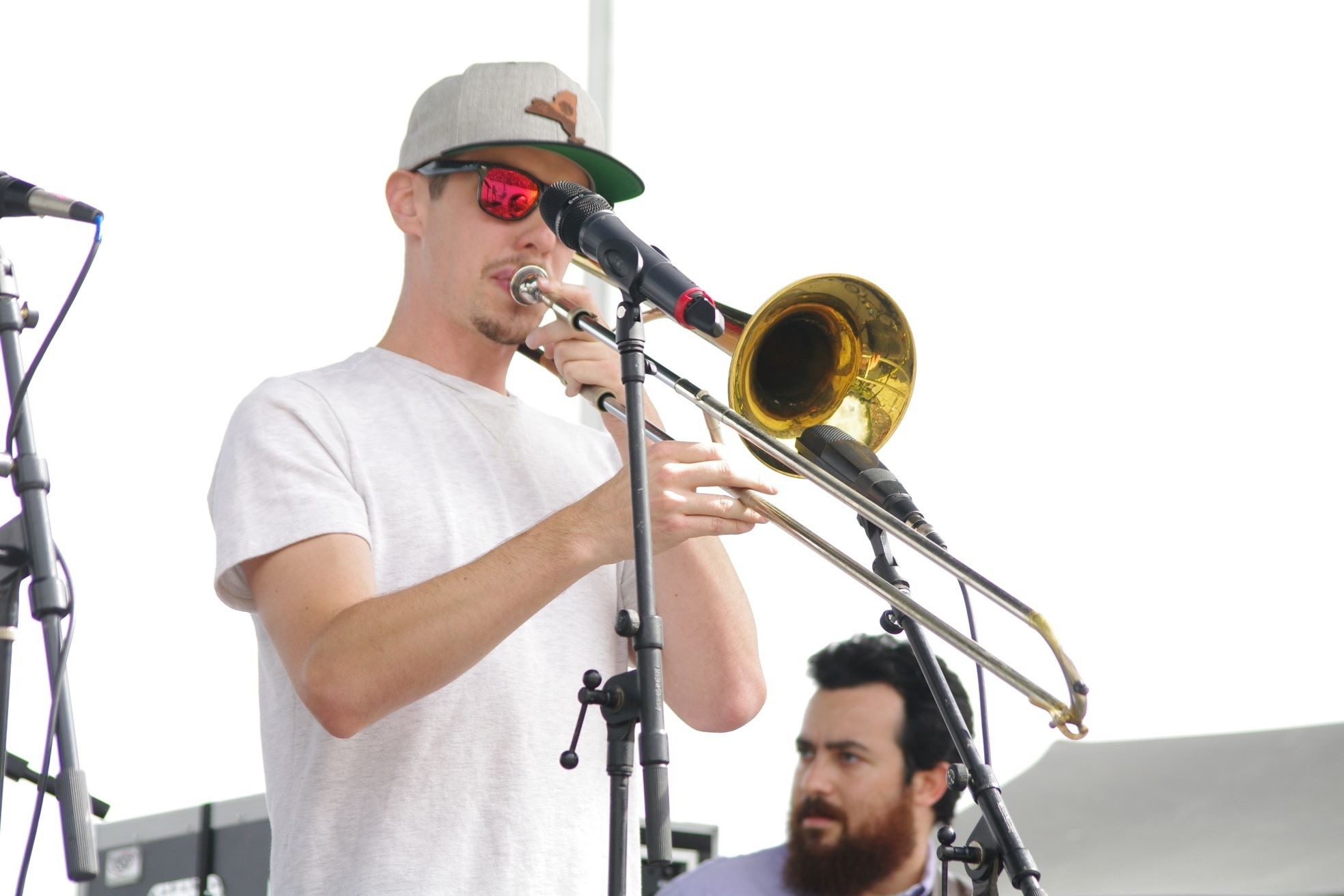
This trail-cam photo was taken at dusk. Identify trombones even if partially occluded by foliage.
[508,249,1093,743]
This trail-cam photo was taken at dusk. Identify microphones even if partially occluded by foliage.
[799,425,947,547]
[541,181,724,337]
[1,170,105,227]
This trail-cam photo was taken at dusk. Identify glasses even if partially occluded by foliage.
[423,160,549,222]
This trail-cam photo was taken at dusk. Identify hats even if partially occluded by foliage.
[391,62,645,203]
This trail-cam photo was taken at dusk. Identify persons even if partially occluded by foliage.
[655,633,973,896]
[206,66,767,896]
